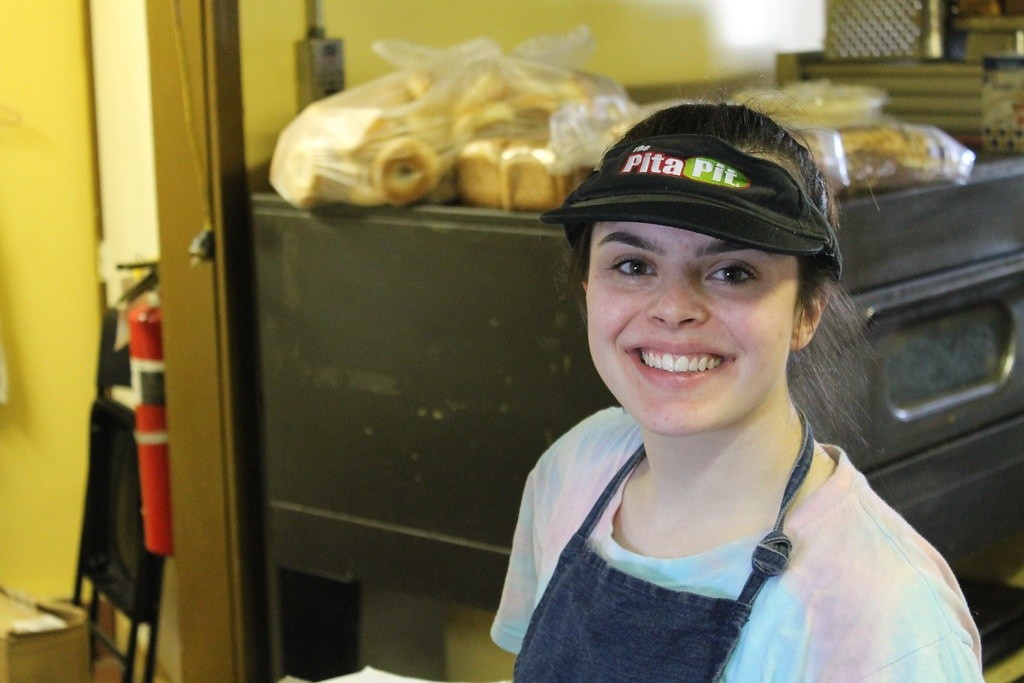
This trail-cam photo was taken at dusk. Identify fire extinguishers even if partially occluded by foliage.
[113,261,175,556]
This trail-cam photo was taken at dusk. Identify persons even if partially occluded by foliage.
[490,102,986,683]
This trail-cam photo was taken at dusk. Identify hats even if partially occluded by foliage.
[537,132,842,282]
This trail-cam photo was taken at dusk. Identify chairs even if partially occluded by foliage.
[70,275,164,683]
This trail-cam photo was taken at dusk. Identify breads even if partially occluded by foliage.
[268,62,961,213]
